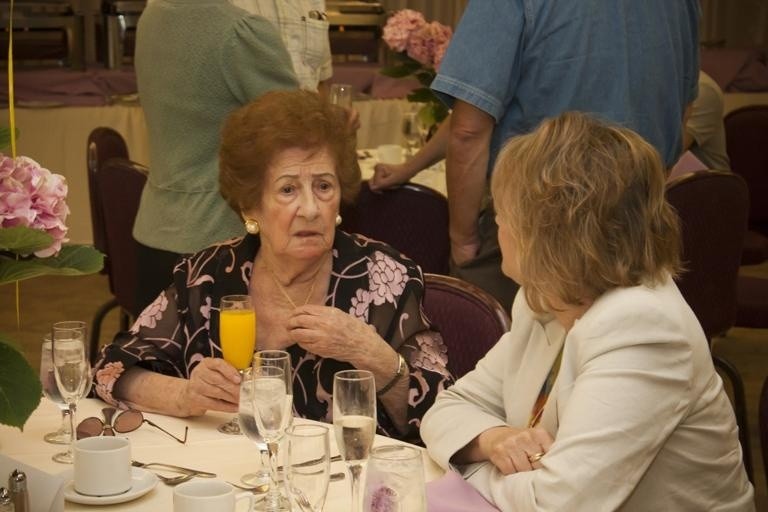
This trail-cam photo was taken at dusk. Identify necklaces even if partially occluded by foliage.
[270,271,323,310]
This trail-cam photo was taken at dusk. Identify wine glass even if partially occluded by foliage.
[39,332,87,445]
[363,444,426,512]
[253,350,294,512]
[217,294,257,435]
[282,424,331,512]
[50,320,90,464]
[332,369,376,512]
[402,111,419,154]
[331,84,351,114]
[239,366,284,491]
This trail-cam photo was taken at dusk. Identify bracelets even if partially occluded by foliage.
[377,355,405,396]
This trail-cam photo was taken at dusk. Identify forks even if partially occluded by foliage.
[154,471,196,485]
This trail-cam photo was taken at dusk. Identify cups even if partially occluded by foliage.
[377,144,402,161]
[173,478,255,512]
[71,437,132,496]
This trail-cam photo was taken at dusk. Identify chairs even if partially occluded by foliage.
[723,102,767,234]
[423,273,511,381]
[88,162,157,367]
[710,355,751,481]
[341,180,451,272]
[86,127,129,299]
[660,169,752,487]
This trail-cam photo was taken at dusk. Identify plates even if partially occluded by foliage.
[64,465,157,505]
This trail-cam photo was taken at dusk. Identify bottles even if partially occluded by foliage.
[0,471,30,511]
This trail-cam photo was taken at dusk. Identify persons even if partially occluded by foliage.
[89,90,455,450]
[369,109,454,193]
[418,112,759,510]
[128,1,300,318]
[681,72,733,172]
[431,1,703,305]
[234,1,334,100]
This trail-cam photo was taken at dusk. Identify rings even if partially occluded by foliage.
[528,451,545,465]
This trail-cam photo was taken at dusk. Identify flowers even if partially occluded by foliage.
[381,6,452,74]
[0,154,109,432]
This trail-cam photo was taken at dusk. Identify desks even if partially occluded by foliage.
[0,96,147,248]
[348,101,444,149]
